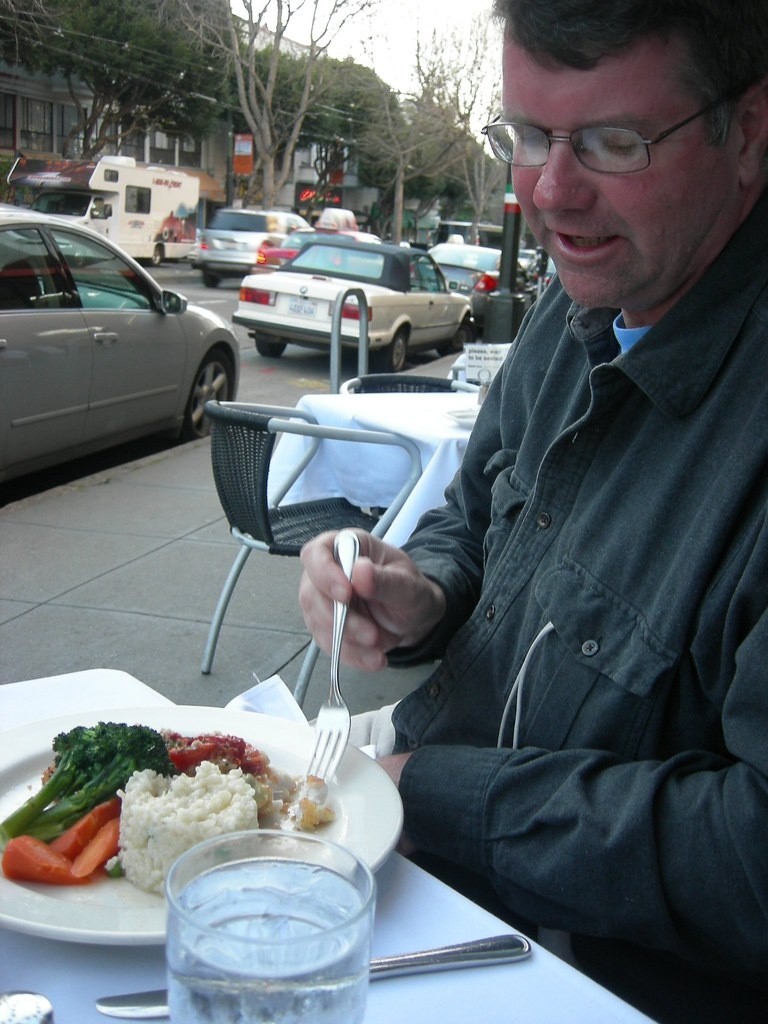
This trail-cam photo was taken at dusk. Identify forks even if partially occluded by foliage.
[304,527,360,780]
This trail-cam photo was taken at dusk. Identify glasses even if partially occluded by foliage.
[481,93,733,174]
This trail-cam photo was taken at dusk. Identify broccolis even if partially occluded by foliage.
[0,721,179,846]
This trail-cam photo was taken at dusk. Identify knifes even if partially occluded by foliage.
[95,934,531,1019]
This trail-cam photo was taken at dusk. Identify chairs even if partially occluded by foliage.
[203,400,424,711]
[0,253,49,309]
[338,374,479,393]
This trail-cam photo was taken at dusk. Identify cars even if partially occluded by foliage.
[0,203,242,484]
[258,208,402,301]
[516,247,539,271]
[417,243,525,337]
[232,243,473,374]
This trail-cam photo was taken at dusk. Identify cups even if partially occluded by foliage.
[165,828,377,1024]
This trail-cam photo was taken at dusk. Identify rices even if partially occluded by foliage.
[114,760,259,896]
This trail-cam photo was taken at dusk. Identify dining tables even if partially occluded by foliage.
[0,667,657,1024]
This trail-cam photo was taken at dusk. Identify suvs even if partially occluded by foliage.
[198,206,314,286]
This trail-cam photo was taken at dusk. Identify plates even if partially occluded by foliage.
[0,706,404,946]
[442,407,481,429]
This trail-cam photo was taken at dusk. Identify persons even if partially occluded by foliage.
[295,0,768,1024]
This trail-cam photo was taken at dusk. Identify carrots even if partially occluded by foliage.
[1,800,125,886]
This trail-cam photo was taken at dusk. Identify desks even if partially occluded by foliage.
[269,392,482,550]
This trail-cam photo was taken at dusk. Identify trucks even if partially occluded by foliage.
[5,150,200,266]
